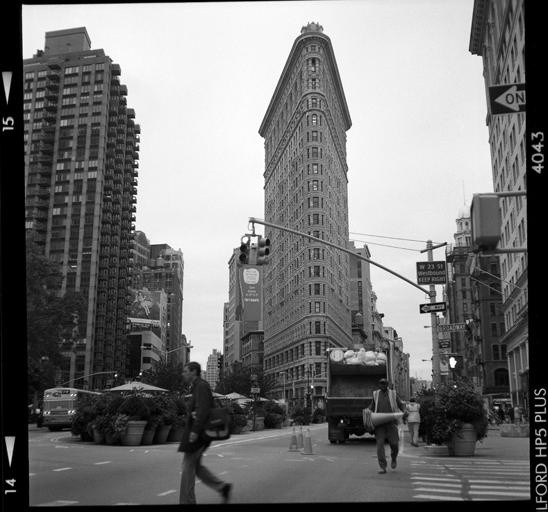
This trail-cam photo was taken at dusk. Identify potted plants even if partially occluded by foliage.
[68,392,285,448]
[410,374,495,459]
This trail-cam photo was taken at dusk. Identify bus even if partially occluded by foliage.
[38,387,101,432]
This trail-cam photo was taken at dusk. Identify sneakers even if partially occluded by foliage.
[391,460,397,469]
[378,470,387,474]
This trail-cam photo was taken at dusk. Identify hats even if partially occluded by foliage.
[378,378,388,385]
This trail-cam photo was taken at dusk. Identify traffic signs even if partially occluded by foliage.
[419,301,447,313]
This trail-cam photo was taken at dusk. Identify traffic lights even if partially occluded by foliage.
[217,354,224,369]
[238,234,271,265]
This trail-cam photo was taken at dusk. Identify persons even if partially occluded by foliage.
[178,360,232,504]
[366,377,408,474]
[484,404,524,426]
[404,397,423,446]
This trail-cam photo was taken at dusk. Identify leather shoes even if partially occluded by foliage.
[219,483,232,504]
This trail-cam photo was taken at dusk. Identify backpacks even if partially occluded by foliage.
[201,408,232,440]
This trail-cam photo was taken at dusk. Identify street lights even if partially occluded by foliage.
[140,345,193,367]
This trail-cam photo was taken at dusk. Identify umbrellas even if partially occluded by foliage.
[103,379,281,404]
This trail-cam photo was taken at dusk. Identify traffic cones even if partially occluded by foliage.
[287,427,300,452]
[300,429,313,455]
[362,407,404,432]
[297,425,305,448]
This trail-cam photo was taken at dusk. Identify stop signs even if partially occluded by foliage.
[448,354,465,371]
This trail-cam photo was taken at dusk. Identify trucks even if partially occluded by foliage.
[324,345,393,444]
